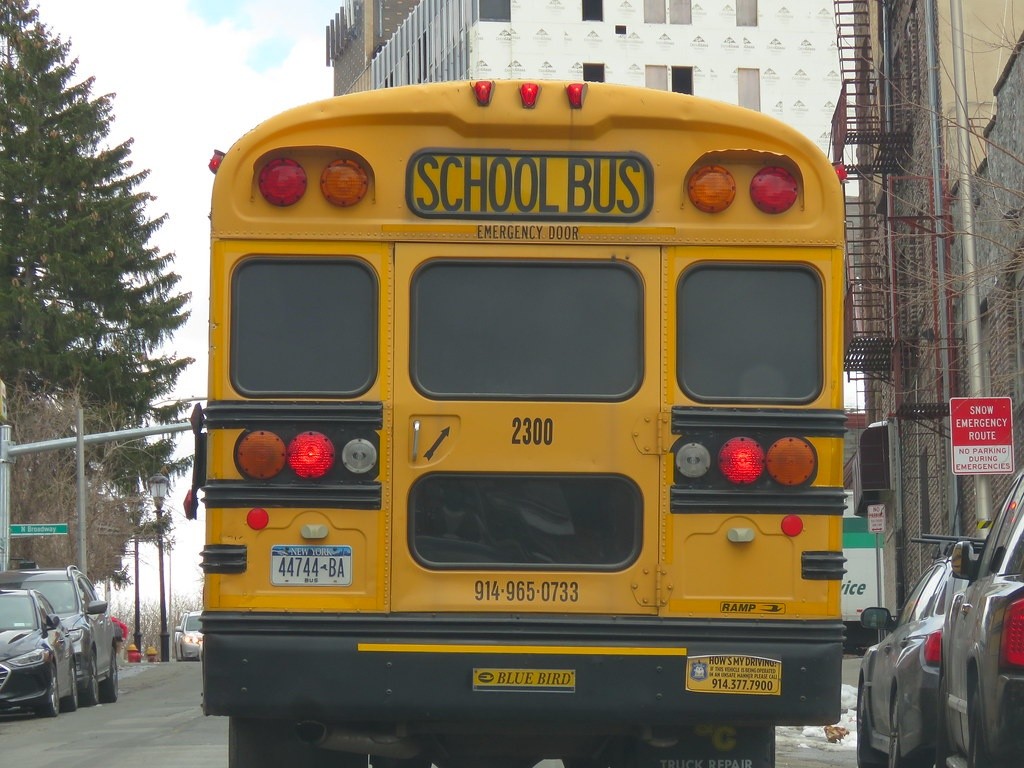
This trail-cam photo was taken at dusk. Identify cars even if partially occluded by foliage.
[0,589,78,720]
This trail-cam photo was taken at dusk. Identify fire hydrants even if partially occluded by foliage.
[127,644,143,662]
[146,647,157,662]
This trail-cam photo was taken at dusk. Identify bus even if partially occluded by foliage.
[177,78,856,768]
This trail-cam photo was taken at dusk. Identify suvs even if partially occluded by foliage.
[857,534,992,768]
[932,465,1024,768]
[174,610,204,662]
[1,563,119,706]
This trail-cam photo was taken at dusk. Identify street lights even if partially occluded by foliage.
[127,495,144,654]
[148,471,172,662]
